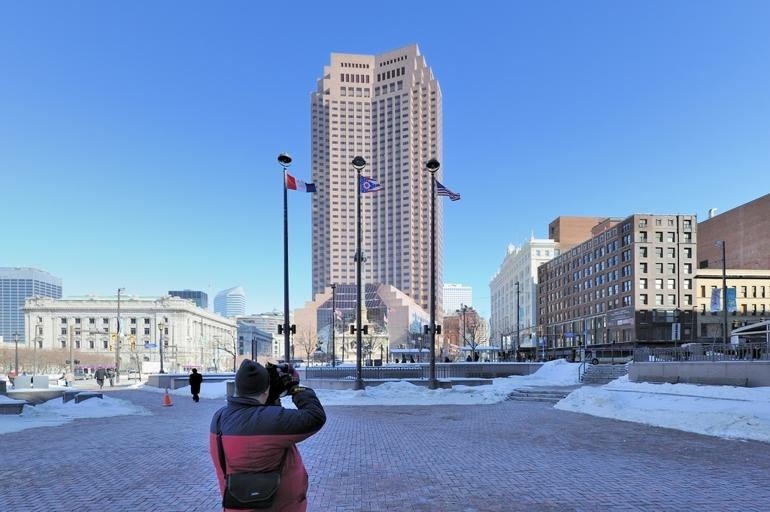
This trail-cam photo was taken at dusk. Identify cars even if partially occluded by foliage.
[208,367,215,371]
[680,342,764,359]
[126,369,140,380]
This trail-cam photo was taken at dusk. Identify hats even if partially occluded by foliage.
[236,359,270,395]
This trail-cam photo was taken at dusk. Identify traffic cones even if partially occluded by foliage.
[159,385,174,407]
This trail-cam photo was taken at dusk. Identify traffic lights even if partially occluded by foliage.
[130,342,135,351]
[109,344,113,352]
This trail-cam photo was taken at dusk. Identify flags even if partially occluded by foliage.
[359,176,386,194]
[433,180,461,202]
[284,172,317,194]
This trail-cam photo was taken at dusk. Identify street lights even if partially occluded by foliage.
[691,306,698,342]
[329,283,337,367]
[424,157,442,391]
[417,336,422,363]
[157,321,165,373]
[672,308,682,360]
[514,281,523,363]
[348,155,370,393]
[113,288,126,383]
[455,308,467,347]
[712,239,728,357]
[276,150,298,366]
[10,330,20,377]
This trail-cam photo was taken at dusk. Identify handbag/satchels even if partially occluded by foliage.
[223,471,280,508]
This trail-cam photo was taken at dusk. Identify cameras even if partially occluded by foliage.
[266,359,298,394]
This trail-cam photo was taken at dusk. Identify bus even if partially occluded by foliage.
[584,347,634,366]
[536,345,586,361]
[182,365,202,371]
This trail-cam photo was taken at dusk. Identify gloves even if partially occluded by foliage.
[289,364,299,393]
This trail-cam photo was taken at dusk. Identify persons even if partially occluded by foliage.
[94,364,108,389]
[188,368,203,402]
[209,359,327,512]
